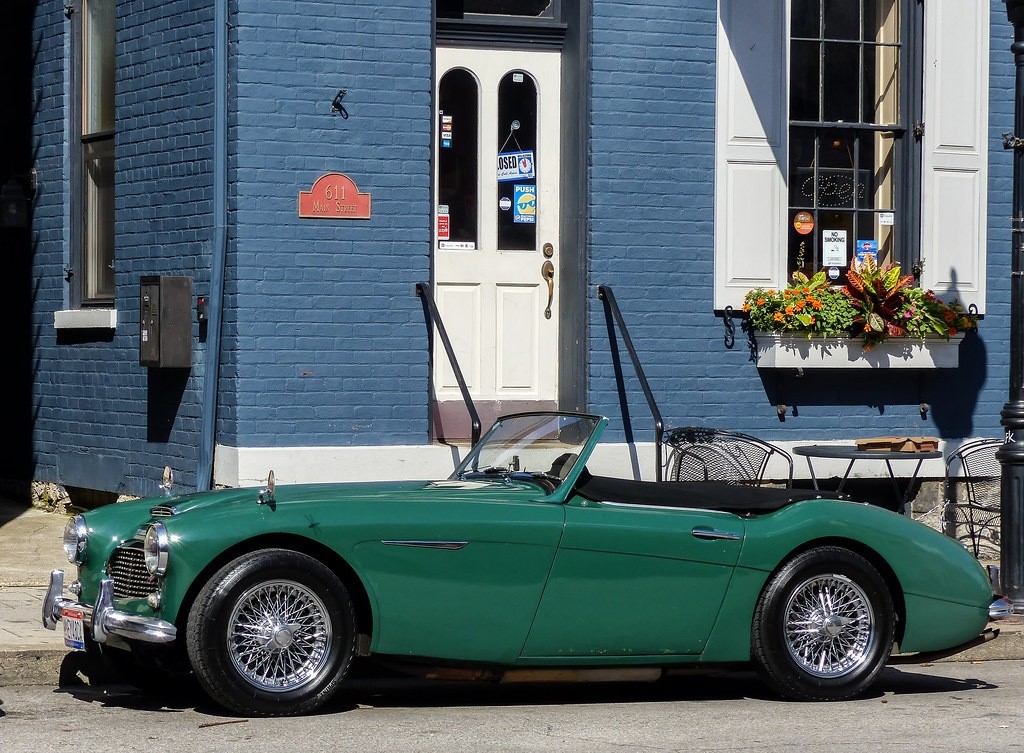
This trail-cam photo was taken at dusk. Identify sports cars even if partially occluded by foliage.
[39,410,1006,718]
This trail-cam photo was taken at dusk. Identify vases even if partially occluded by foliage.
[753,329,965,369]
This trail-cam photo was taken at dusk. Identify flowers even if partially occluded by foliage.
[740,253,972,343]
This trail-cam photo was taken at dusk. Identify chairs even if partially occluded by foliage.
[941,438,1006,559]
[658,426,792,490]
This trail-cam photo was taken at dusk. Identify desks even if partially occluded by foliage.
[792,445,943,513]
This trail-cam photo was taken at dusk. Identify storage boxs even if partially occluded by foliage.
[856,435,939,453]
